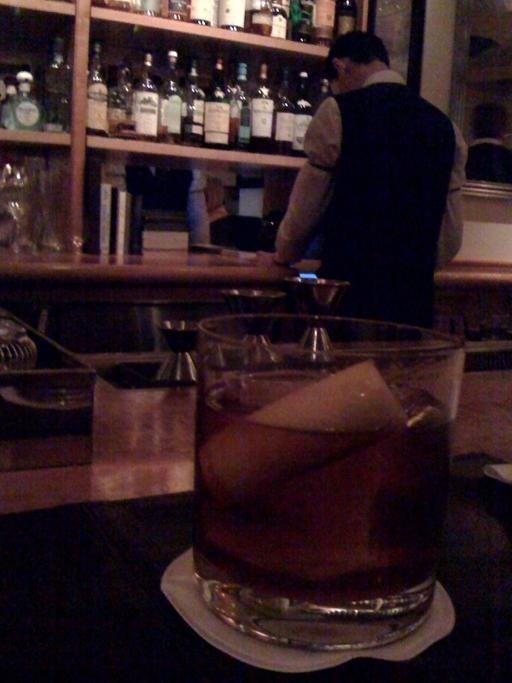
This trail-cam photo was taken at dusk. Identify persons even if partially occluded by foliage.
[465,99,511,185]
[272,29,463,335]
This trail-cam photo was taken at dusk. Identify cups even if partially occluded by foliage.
[191,312,469,654]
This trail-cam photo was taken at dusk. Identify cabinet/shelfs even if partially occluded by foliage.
[0,0,370,352]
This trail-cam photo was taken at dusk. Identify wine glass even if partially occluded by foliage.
[0,162,42,254]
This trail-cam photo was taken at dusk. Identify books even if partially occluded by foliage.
[143,225,189,249]
[88,159,131,255]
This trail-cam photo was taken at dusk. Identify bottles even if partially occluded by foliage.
[235,165,267,253]
[1,0,356,158]
